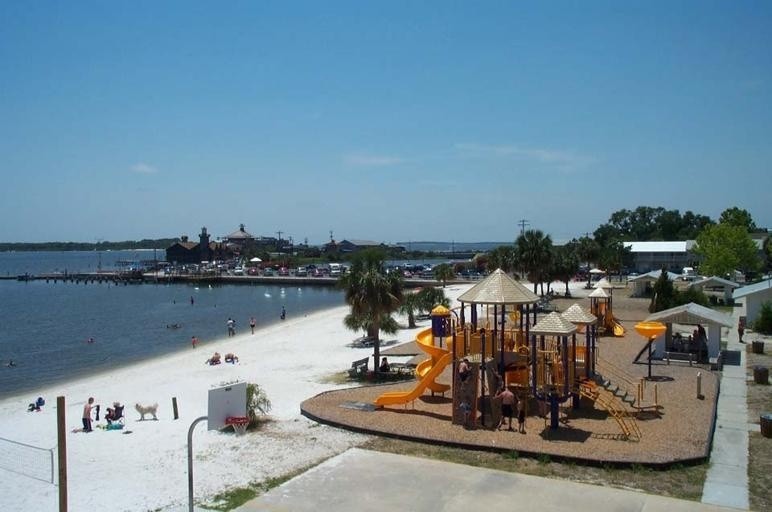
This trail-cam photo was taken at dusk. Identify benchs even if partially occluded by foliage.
[348,357,369,377]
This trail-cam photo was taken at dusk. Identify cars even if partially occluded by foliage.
[296,267,308,276]
[125,258,237,275]
[264,268,274,276]
[307,264,329,277]
[385,262,432,278]
[248,267,258,275]
[278,267,289,276]
[234,266,243,275]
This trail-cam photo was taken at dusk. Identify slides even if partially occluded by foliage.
[375,351,453,406]
[414,327,451,392]
[577,308,594,333]
[605,310,624,336]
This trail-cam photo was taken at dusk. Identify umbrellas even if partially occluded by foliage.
[589,267,606,281]
[249,256,262,262]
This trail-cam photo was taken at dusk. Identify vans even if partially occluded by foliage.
[329,264,342,277]
[682,267,693,281]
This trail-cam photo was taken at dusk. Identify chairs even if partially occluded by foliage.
[114,410,125,426]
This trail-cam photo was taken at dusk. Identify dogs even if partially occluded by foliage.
[134,402,159,421]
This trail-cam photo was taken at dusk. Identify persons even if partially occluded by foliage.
[190,335,197,350]
[280,304,287,320]
[491,367,506,395]
[226,316,235,337]
[514,394,528,434]
[736,318,745,343]
[204,351,239,366]
[249,315,257,334]
[458,394,473,428]
[105,402,123,426]
[670,323,707,352]
[457,358,474,391]
[490,385,516,431]
[378,356,390,371]
[70,396,97,434]
